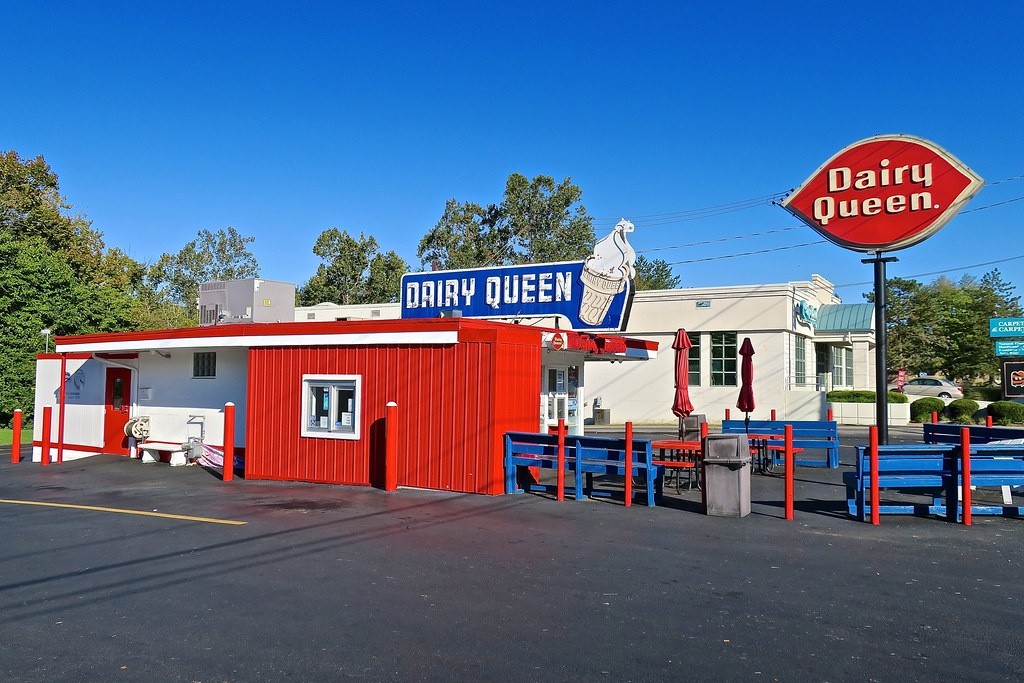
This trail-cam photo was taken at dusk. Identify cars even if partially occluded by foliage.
[888,375,964,400]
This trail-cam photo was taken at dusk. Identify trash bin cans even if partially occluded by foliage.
[594,408,610,426]
[703,432,752,518]
[683,414,707,441]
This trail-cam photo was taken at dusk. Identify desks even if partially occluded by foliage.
[651,440,702,492]
[749,433,785,476]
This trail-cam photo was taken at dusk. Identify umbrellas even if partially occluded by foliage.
[736,338,755,434]
[672,328,695,440]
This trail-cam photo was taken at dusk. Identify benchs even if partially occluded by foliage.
[749,444,804,472]
[503,431,666,508]
[652,461,695,496]
[923,422,1024,445]
[721,420,839,469]
[676,449,759,475]
[842,444,1024,521]
[137,442,187,466]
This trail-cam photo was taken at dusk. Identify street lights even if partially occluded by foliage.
[40,329,51,354]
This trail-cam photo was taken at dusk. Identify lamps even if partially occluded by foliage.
[150,350,171,358]
